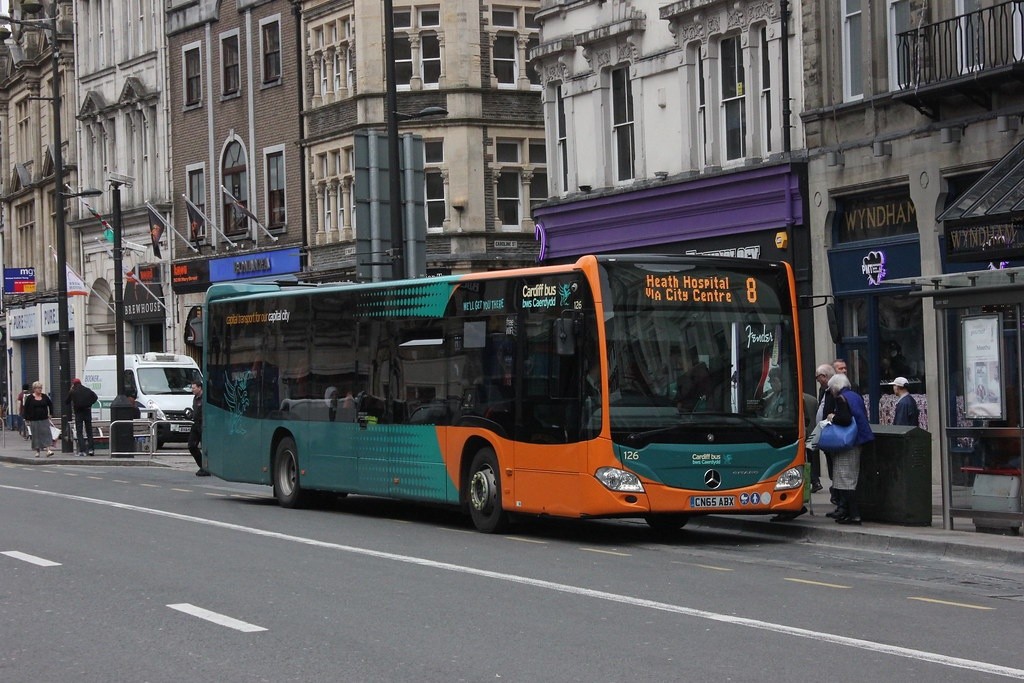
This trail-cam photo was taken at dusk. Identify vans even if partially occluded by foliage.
[81,352,203,446]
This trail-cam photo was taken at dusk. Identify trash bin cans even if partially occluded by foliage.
[135,407,158,453]
[852,424,933,526]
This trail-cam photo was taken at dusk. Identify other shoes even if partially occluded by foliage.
[89,449,94,455]
[826,508,861,525]
[47,450,54,457]
[35,451,40,456]
[76,451,86,457]
[195,468,209,476]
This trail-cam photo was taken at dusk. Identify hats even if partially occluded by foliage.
[888,377,909,388]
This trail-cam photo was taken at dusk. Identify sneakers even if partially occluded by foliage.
[810,482,823,493]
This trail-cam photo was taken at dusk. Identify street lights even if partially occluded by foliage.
[0,11,103,453]
[388,106,449,280]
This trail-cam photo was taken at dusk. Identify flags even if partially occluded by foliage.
[147,205,165,260]
[78,196,126,245]
[186,201,203,254]
[54,254,88,297]
[222,190,258,223]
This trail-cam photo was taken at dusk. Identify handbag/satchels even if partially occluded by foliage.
[818,394,858,451]
[48,418,60,441]
[803,450,811,502]
[805,419,832,452]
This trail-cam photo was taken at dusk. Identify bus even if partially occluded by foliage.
[202,254,842,533]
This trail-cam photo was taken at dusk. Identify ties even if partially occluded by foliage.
[815,373,824,380]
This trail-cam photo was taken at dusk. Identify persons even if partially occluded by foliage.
[641,351,872,525]
[24,381,54,457]
[887,377,920,426]
[188,380,210,476]
[127,395,146,445]
[0,384,30,435]
[64,378,95,456]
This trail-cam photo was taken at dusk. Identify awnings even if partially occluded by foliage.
[935,140,1024,224]
